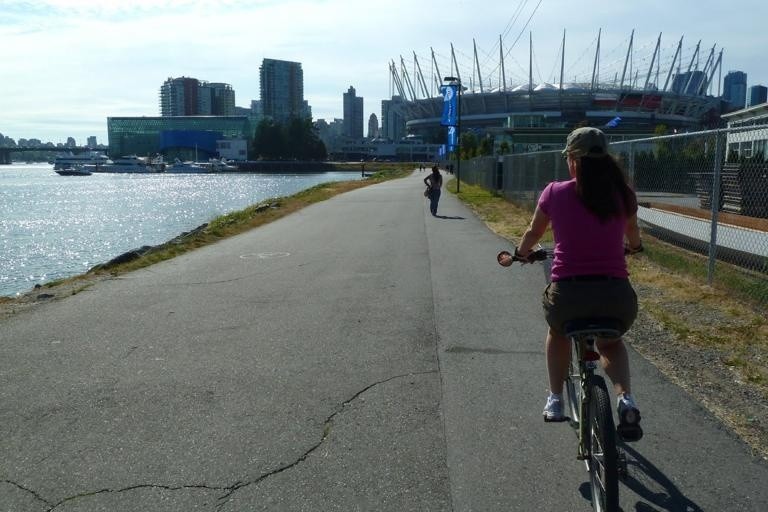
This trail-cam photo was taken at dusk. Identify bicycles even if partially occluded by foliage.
[497,247,644,512]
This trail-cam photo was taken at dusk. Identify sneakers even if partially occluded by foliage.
[542,397,564,421]
[616,390,642,442]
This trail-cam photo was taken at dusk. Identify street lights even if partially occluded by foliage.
[444,76,462,192]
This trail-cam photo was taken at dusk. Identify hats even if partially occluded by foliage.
[562,126,608,158]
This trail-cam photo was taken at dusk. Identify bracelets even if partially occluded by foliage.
[513,245,524,258]
[627,241,642,251]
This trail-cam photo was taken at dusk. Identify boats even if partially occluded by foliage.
[54,154,239,176]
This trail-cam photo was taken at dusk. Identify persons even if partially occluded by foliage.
[422,164,425,172]
[449,161,455,176]
[419,164,421,172]
[510,125,643,443]
[422,164,443,216]
[444,163,448,174]
